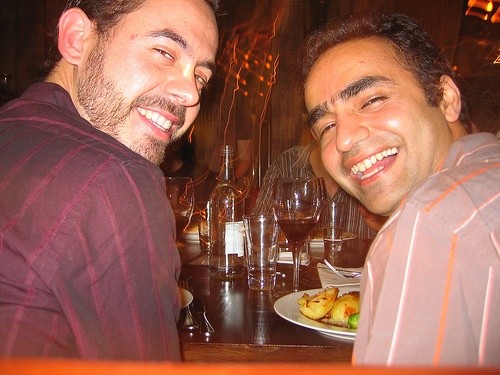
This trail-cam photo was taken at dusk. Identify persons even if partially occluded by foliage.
[1,1,220,362]
[165,12,500,368]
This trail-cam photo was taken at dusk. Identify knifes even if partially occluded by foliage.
[188,290,215,337]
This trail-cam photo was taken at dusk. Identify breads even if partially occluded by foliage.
[296,287,339,320]
[322,291,361,327]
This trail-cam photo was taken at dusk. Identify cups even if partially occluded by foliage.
[195,201,210,255]
[320,202,346,252]
[242,215,280,291]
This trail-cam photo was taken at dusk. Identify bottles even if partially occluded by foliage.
[206,144,246,279]
[271,177,327,299]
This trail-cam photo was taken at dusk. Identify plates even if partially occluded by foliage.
[309,231,357,247]
[273,288,361,340]
[179,287,193,310]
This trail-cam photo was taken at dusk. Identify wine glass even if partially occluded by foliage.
[164,177,194,245]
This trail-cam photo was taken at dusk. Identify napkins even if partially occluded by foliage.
[316,261,364,289]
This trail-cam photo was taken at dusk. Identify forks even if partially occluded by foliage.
[177,280,200,330]
